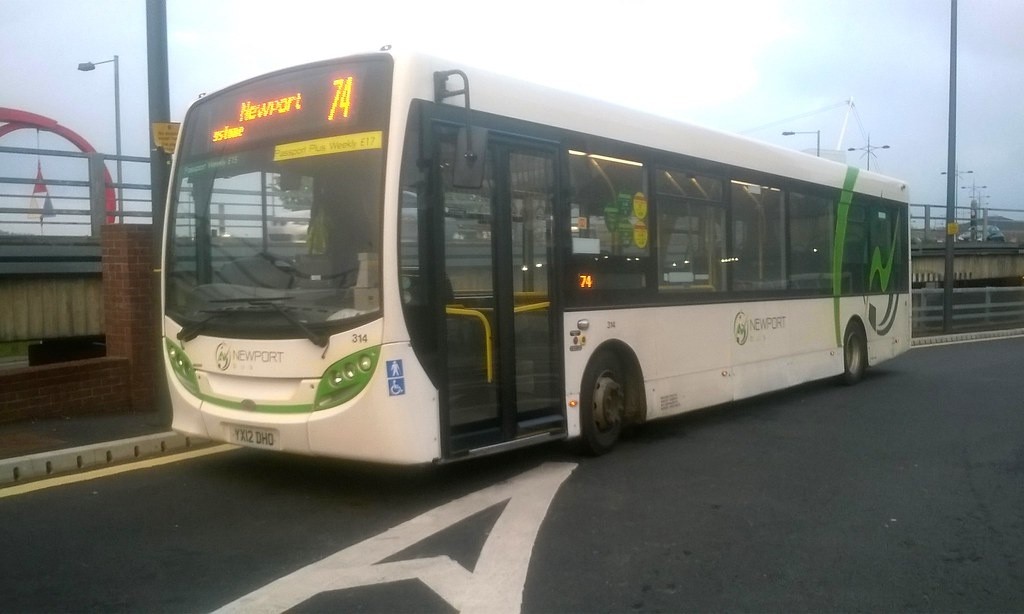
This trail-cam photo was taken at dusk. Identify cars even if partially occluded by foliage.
[958,224,1007,243]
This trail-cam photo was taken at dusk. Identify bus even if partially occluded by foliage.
[159,46,916,470]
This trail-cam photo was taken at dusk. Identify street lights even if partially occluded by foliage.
[941,167,974,224]
[78,56,124,227]
[847,134,891,172]
[961,185,991,218]
[780,130,821,158]
